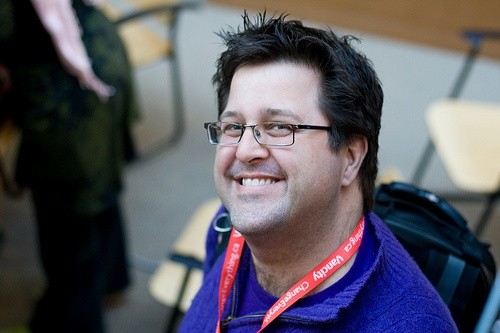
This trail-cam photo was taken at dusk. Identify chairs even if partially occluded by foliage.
[94,0,201,163]
[405,25,499,253]
[148,166,405,333]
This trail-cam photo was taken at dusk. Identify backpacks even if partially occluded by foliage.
[371,181,497,333]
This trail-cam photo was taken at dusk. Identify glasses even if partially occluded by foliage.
[203,120,332,146]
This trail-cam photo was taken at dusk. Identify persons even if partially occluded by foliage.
[177,5,459,333]
[0,0,146,333]
